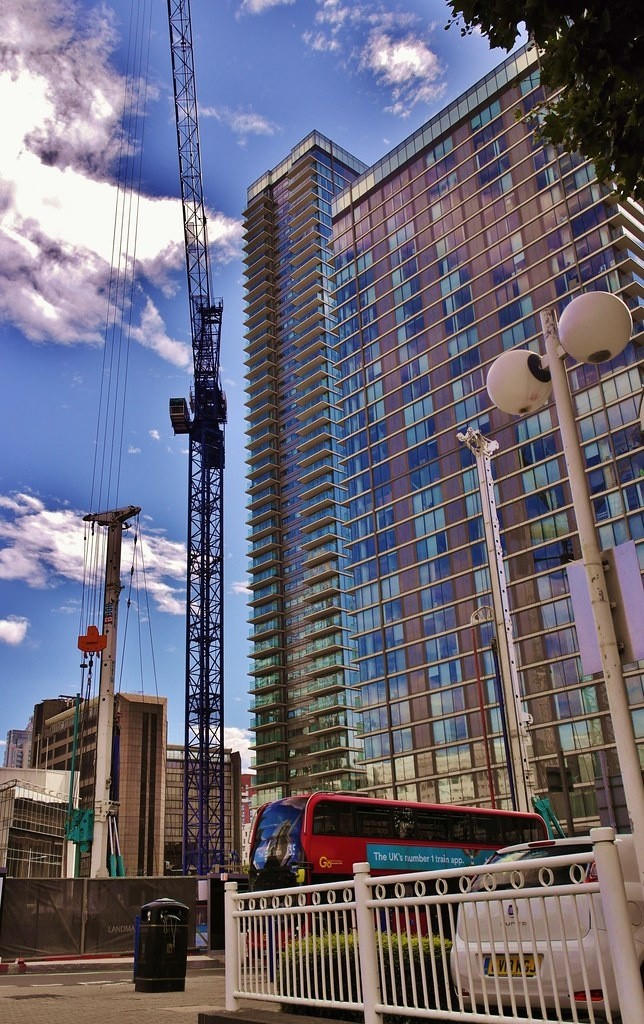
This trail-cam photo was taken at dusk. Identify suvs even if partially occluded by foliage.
[448,832,644,1017]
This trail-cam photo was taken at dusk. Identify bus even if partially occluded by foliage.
[246,788,548,979]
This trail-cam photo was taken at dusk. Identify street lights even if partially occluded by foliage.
[485,291,644,881]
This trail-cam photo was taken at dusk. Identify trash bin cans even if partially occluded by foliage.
[133,897,191,994]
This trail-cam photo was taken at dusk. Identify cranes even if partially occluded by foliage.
[64,0,237,878]
